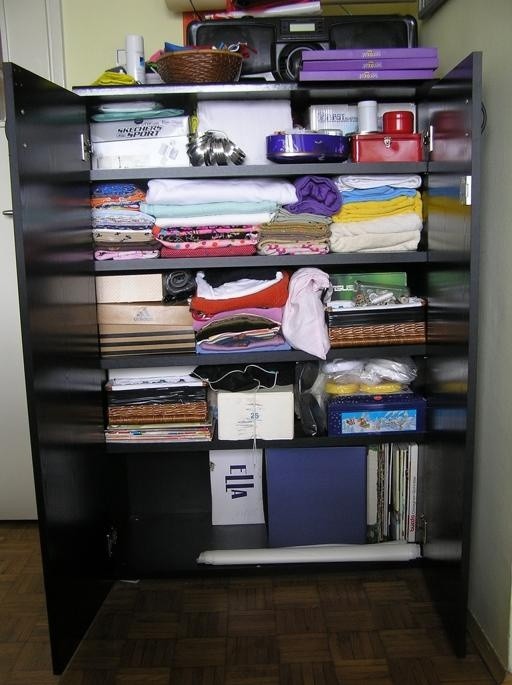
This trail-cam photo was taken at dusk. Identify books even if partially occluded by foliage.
[366,442,421,541]
[104,412,219,444]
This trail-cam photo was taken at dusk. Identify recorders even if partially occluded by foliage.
[186,14,419,84]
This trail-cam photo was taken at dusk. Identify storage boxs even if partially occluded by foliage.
[265,447,366,547]
[216,383,295,441]
[352,133,421,163]
[197,99,292,165]
[325,391,427,437]
[89,116,193,169]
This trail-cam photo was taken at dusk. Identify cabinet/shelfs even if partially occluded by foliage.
[3,51,482,677]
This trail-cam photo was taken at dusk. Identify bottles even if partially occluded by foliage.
[125,35,145,83]
[357,100,378,133]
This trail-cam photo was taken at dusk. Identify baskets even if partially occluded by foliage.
[156,50,243,84]
